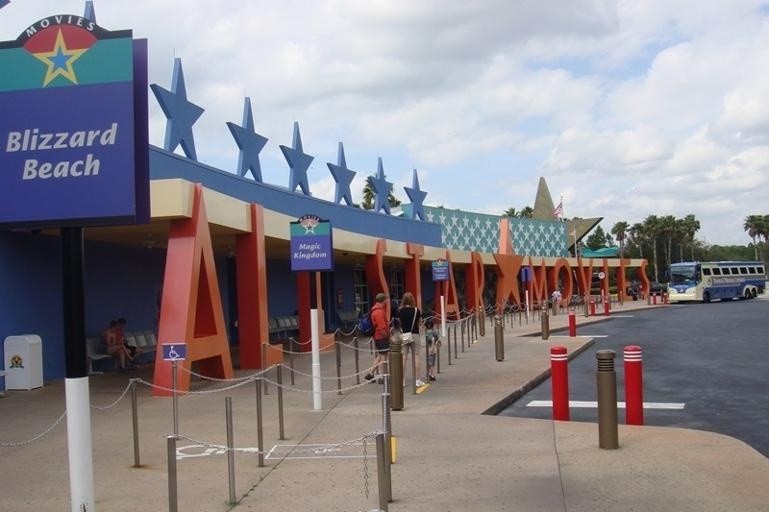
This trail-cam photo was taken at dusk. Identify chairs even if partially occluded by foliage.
[336,310,360,327]
[267,315,300,338]
[85,330,157,376]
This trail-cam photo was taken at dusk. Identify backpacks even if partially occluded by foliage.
[358,307,383,337]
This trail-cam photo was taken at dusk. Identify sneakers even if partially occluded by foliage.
[365,371,435,384]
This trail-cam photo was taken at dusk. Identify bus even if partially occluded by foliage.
[665,261,765,302]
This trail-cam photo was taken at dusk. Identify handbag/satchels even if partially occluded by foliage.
[400,331,415,347]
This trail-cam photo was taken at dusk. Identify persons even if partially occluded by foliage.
[398,291,426,387]
[388,317,403,338]
[552,287,562,316]
[626,279,641,300]
[364,293,390,385]
[103,319,134,374]
[356,294,463,318]
[117,317,144,367]
[425,318,441,381]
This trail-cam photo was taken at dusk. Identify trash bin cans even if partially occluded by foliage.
[4,335,43,390]
[633,292,638,300]
